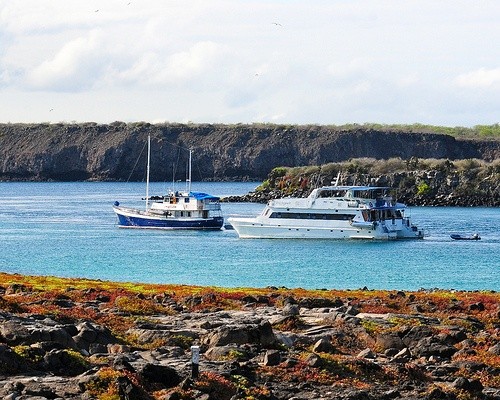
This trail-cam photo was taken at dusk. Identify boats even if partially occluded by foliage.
[228,172,425,240]
[111,136,224,229]
[450,234,482,240]
